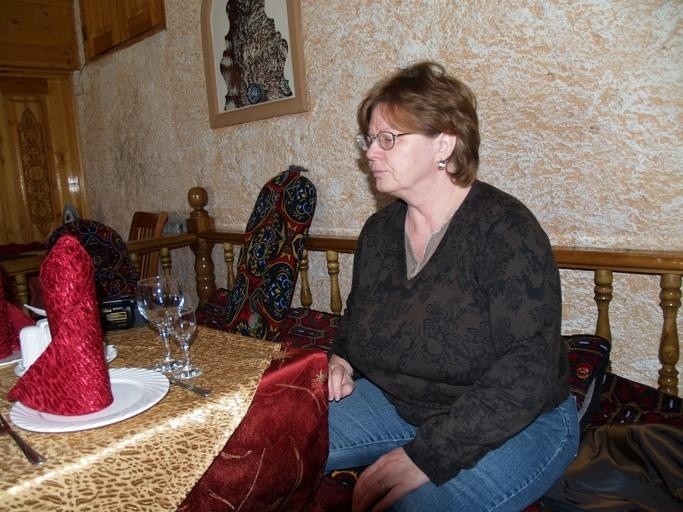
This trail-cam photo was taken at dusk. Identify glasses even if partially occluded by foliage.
[355,131,414,151]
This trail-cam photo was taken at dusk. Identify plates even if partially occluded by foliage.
[14,345,119,377]
[8,367,171,433]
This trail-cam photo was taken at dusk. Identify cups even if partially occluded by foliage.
[18,326,46,368]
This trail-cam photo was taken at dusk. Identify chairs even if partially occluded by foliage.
[125,209,172,304]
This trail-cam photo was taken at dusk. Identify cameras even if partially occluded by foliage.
[100,296,150,330]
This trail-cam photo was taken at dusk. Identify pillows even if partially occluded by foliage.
[224,165,319,342]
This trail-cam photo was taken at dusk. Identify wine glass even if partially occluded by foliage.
[165,305,205,380]
[136,275,185,373]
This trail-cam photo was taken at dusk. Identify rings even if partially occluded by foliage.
[378,479,387,492]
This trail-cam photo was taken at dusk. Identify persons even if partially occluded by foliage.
[319,60,579,512]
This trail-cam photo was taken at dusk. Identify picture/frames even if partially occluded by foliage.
[200,0,310,129]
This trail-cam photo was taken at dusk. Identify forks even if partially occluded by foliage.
[0,413,46,465]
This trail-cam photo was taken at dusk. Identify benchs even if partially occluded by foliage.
[177,186,683,512]
